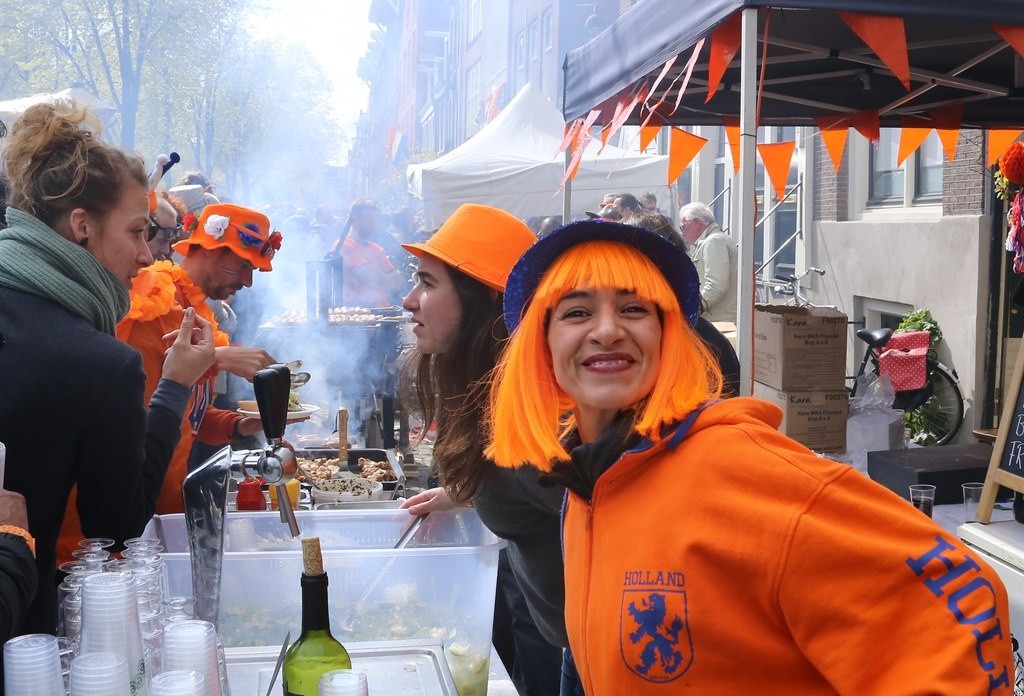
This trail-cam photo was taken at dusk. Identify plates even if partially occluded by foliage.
[236,403,321,420]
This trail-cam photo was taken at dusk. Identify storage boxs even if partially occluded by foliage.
[749,304,848,393]
[139,506,508,696]
[749,379,850,455]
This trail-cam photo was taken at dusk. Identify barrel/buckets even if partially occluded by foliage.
[312,478,383,502]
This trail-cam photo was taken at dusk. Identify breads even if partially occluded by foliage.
[238,400,258,412]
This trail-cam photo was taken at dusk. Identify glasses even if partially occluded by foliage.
[230,222,272,258]
[679,218,697,234]
[148,211,182,237]
[147,222,158,242]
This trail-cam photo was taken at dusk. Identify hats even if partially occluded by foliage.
[400,202,538,293]
[586,204,621,221]
[502,217,701,336]
[168,186,208,216]
[171,203,273,272]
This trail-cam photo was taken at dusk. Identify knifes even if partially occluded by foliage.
[337,408,350,471]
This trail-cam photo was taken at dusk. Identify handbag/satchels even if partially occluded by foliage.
[876,330,932,414]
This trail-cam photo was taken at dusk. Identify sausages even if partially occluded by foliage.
[274,306,377,326]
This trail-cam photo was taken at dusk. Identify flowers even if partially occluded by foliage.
[269,228,283,254]
[182,209,202,233]
[202,214,231,241]
[236,221,263,248]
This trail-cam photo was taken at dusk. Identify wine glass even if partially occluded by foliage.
[44,537,229,695]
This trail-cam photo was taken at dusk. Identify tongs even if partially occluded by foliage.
[279,359,311,388]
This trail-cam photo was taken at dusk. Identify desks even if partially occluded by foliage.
[859,441,1015,539]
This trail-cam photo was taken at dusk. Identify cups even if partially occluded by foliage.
[318,665,369,696]
[5,571,227,696]
[961,481,985,522]
[909,483,936,521]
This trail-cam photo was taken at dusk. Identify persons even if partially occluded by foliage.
[677,202,739,324]
[483,219,1015,696]
[0,95,425,696]
[527,192,688,252]
[402,205,566,696]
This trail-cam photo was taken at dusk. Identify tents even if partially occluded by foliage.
[407,82,677,232]
[563,0,1024,397]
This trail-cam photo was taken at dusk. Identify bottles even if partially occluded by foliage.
[281,571,354,696]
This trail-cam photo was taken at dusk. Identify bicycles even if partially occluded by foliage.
[757,267,966,449]
